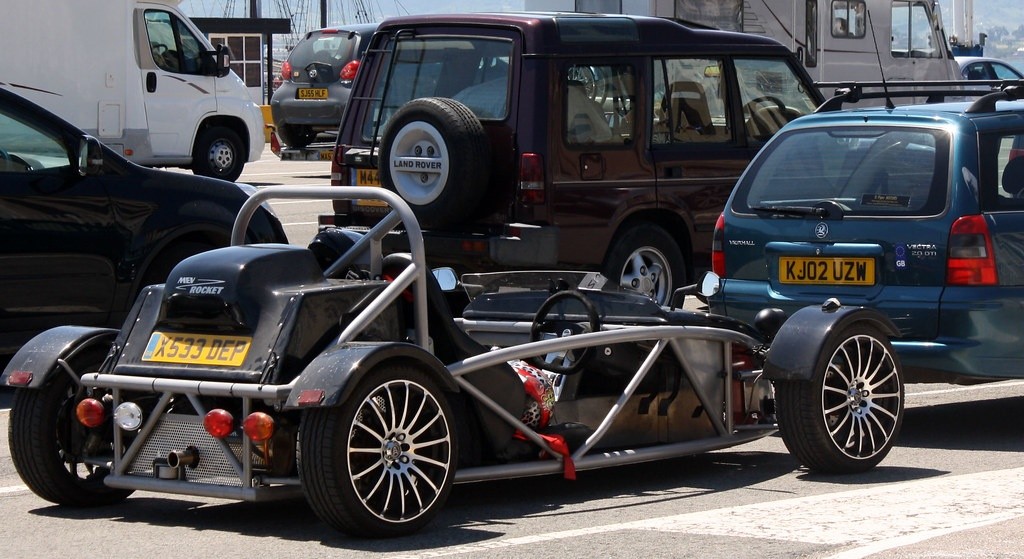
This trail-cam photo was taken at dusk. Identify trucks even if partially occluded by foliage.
[0,0,265,188]
[524,0,966,129]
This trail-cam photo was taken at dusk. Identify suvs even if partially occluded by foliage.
[704,77,1024,408]
[330,13,863,310]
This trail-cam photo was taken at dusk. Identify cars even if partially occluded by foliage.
[944,55,1023,106]
[0,88,289,387]
[269,22,384,149]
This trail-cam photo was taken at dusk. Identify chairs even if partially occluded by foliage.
[567,80,624,143]
[967,70,983,80]
[1001,155,1024,200]
[660,81,717,136]
[306,228,372,279]
[833,18,854,37]
